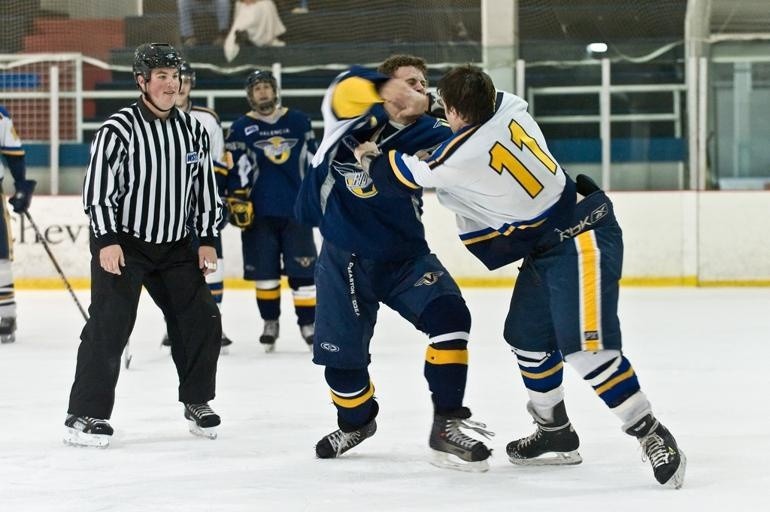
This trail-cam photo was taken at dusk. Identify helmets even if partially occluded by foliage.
[246,70,280,113]
[133,41,196,91]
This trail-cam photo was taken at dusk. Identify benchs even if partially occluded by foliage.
[0,0,685,139]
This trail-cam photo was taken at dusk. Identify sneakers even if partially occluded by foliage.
[184,402,221,428]
[259,320,279,344]
[1,317,16,334]
[300,324,315,347]
[430,414,489,461]
[316,420,377,456]
[65,411,113,436]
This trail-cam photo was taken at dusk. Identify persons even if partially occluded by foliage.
[353,65,681,484]
[293,57,496,463]
[178,0,231,63]
[223,0,286,66]
[62,41,224,434]
[225,70,320,344]
[290,0,309,15]
[0,114,36,334]
[162,60,232,345]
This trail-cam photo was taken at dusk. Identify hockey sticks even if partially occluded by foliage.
[125,339,132,369]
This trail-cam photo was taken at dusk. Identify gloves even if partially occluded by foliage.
[9,180,38,214]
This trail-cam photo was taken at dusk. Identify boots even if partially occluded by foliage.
[620,401,680,485]
[506,400,579,459]
